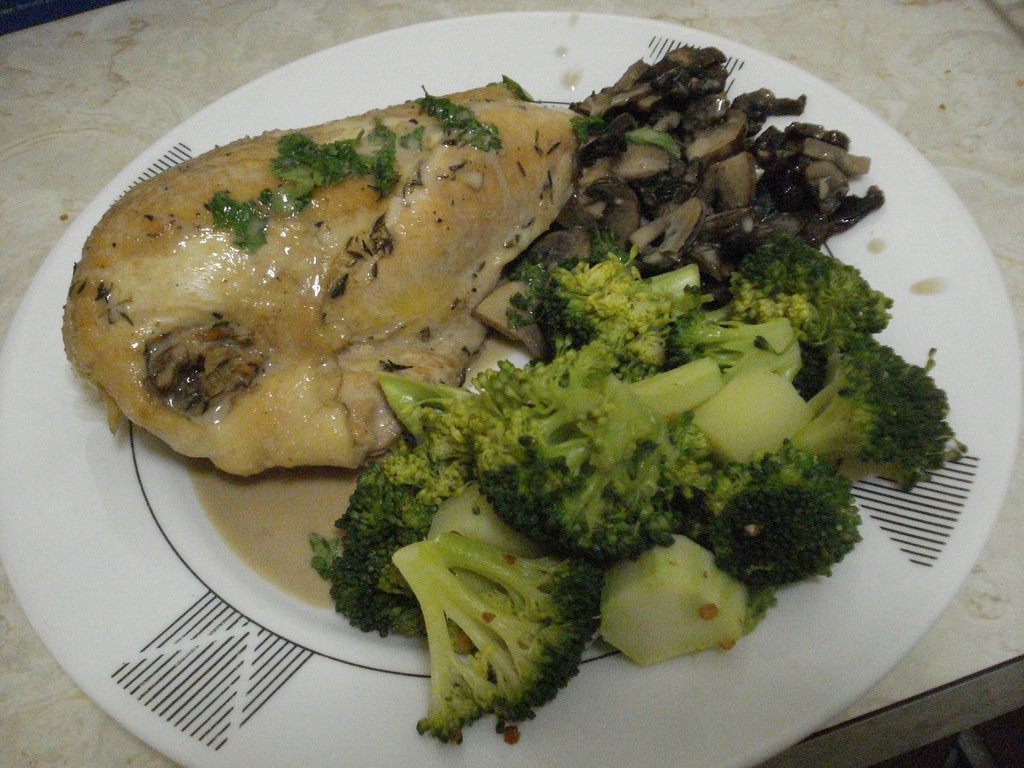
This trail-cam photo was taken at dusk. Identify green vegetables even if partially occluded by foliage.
[206,72,685,251]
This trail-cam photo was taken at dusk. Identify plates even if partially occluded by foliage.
[1,7,1024,767]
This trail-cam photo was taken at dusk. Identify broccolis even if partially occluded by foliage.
[301,222,971,745]
